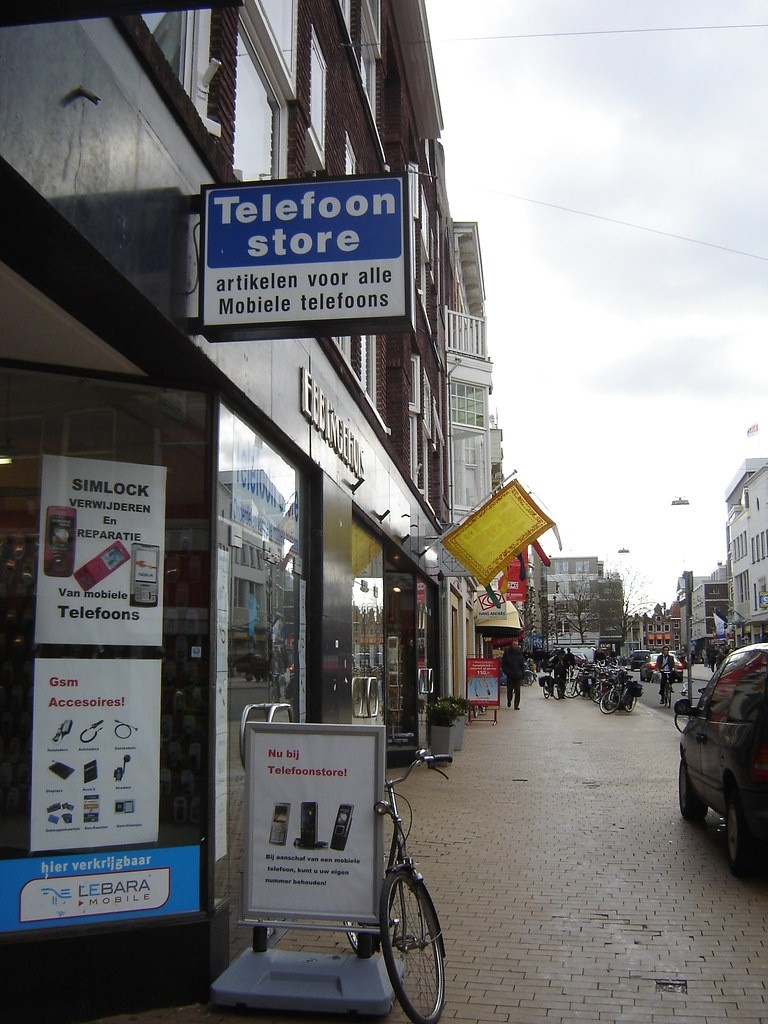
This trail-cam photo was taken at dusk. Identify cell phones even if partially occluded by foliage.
[44,506,80,578]
[129,542,159,606]
[73,540,130,591]
[301,802,319,846]
[269,802,292,845]
[331,803,354,852]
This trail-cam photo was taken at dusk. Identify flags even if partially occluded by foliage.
[442,478,562,608]
[712,607,728,639]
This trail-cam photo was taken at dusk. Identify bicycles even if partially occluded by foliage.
[268,672,280,705]
[674,680,708,734]
[521,670,538,687]
[342,747,454,1024]
[543,660,639,715]
[661,670,675,708]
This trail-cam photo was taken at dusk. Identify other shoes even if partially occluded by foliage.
[660,699,663,704]
[507,702,511,707]
[670,690,674,693]
[514,707,520,710]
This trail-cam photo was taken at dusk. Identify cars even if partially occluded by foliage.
[674,643,768,880]
[640,654,683,683]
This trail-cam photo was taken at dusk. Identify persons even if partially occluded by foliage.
[691,640,739,672]
[656,646,678,704]
[594,644,613,665]
[501,638,525,710]
[243,645,295,703]
[524,647,576,699]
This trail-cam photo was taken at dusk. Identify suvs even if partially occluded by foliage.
[630,649,650,672]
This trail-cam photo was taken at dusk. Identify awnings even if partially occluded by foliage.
[475,592,526,632]
